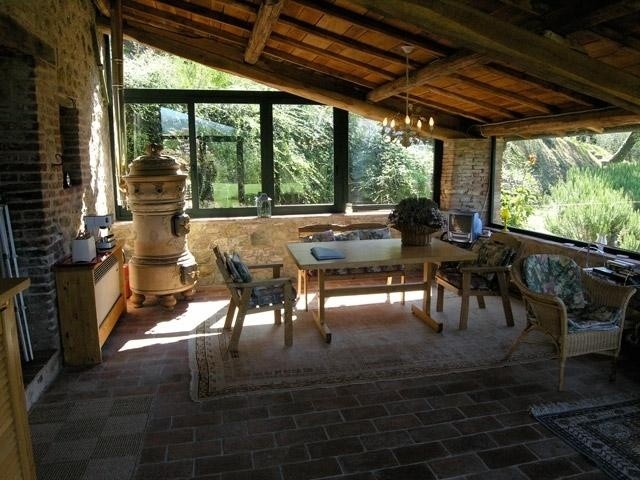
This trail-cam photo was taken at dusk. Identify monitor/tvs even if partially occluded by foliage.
[447,209,483,244]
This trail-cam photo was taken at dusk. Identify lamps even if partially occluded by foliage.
[378,46,434,149]
[388,198,447,233]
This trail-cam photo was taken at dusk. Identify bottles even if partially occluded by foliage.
[257,193,272,217]
[345,203,353,214]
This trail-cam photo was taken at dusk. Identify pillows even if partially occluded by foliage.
[227,251,254,296]
[523,253,584,308]
[299,227,392,242]
[457,237,510,280]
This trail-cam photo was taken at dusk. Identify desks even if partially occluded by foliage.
[286,238,479,343]
[54,244,127,365]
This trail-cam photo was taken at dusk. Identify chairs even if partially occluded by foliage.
[213,245,297,352]
[435,233,521,330]
[500,253,637,391]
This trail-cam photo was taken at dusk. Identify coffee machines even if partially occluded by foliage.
[83,213,116,251]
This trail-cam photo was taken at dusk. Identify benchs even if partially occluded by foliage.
[298,223,405,311]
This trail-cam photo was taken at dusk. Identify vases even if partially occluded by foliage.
[401,232,432,245]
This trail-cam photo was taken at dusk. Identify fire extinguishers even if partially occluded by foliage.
[121,249,130,299]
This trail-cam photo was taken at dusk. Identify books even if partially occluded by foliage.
[593,257,640,286]
[310,246,346,260]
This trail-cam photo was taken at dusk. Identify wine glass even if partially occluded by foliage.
[594,232,608,254]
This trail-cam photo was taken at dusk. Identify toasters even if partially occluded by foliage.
[71,235,97,265]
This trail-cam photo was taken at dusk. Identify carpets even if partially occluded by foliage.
[188,280,560,402]
[527,390,640,480]
